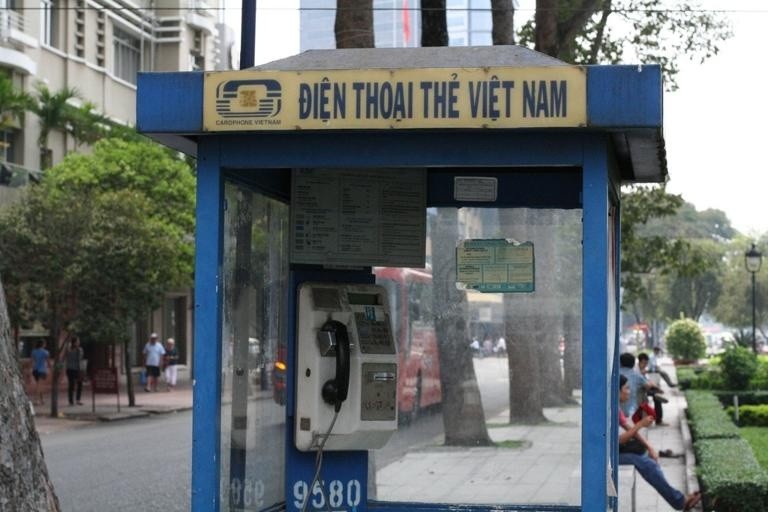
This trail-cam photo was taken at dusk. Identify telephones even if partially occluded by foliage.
[294,282,398,452]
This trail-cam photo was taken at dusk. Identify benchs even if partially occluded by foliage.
[646,372,660,388]
[616,427,649,512]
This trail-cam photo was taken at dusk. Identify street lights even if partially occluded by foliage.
[745,244,762,354]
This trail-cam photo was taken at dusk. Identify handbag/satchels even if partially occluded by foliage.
[619,440,645,454]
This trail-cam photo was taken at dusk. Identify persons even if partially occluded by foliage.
[469,332,508,356]
[161,337,179,389]
[27,339,53,405]
[143,332,167,393]
[618,373,701,511]
[631,353,670,427]
[639,346,679,388]
[619,353,654,420]
[59,336,86,406]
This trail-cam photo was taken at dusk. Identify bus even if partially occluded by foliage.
[377,265,472,427]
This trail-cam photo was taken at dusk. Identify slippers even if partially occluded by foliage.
[682,492,702,511]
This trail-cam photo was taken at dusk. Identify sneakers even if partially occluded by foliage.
[654,393,668,402]
